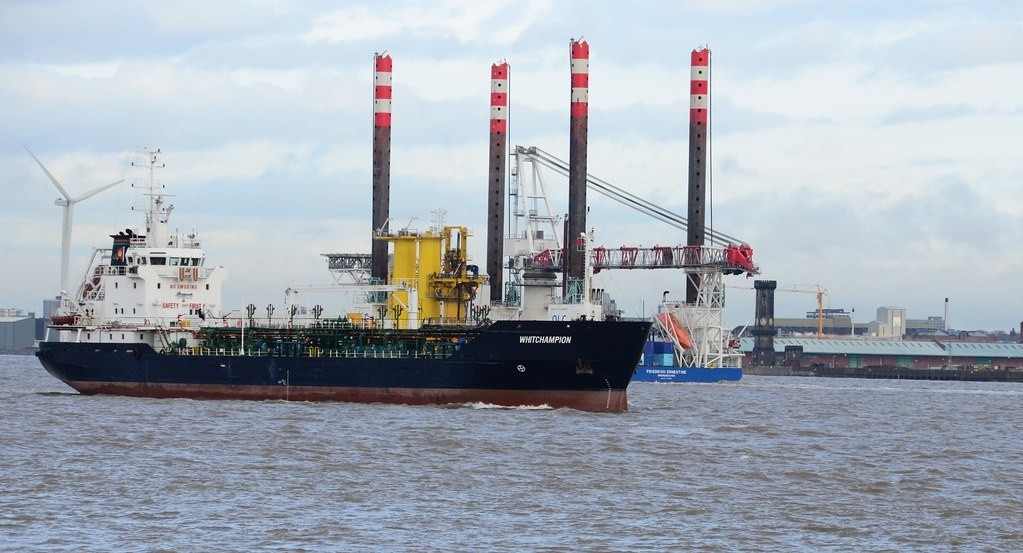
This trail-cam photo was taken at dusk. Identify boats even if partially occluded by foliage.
[318,33,742,385]
[35,145,656,414]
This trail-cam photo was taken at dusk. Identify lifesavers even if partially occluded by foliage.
[116,248,125,259]
[160,349,165,355]
[145,320,151,326]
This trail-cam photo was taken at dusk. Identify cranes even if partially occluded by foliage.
[724,281,831,339]
[505,143,763,317]
